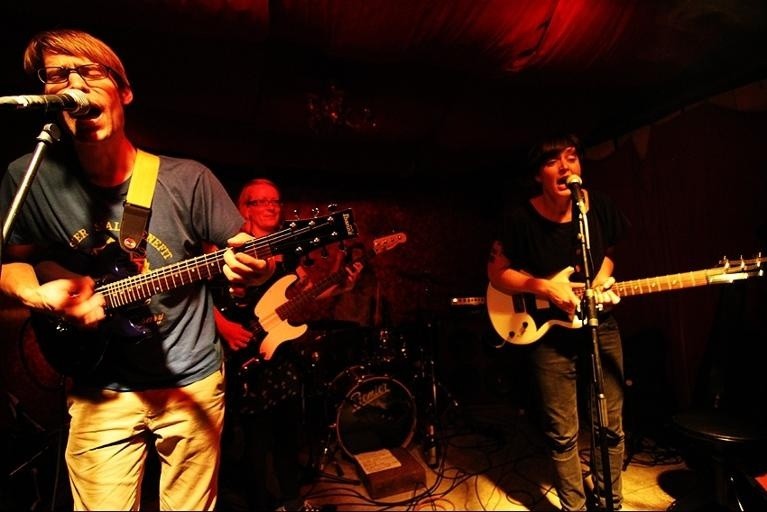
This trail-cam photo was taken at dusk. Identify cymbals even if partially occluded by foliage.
[394,272,445,284]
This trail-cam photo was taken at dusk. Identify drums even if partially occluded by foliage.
[324,365,416,457]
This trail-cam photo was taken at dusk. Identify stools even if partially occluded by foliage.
[672,409,764,505]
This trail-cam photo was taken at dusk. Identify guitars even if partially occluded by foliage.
[487,252,767,345]
[221,232,406,374]
[0,203,358,479]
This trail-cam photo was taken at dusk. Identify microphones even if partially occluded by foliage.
[564,173,588,217]
[0,87,92,118]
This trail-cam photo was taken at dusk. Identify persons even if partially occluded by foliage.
[486,129,627,512]
[212,176,365,511]
[0,27,277,512]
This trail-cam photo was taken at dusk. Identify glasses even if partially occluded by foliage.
[36,62,109,85]
[245,197,285,208]
